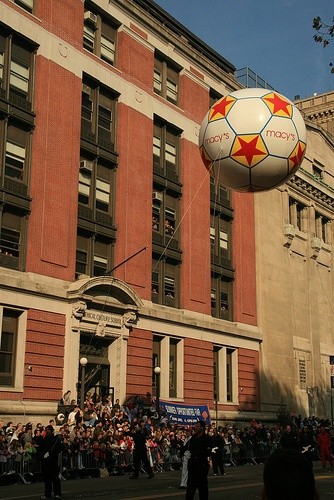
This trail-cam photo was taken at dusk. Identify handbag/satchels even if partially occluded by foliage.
[43,451,50,459]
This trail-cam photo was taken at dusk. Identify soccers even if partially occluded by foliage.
[198,88,308,194]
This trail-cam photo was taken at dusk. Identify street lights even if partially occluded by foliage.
[79,357,88,409]
[154,366,161,417]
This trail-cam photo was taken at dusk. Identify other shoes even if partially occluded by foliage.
[53,495,63,500]
[212,474,218,476]
[40,496,50,500]
[147,474,155,479]
[130,475,138,479]
[223,472,226,476]
[177,485,187,489]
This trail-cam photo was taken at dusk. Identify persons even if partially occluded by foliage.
[0,390,277,475]
[263,432,320,500]
[36,425,63,500]
[277,408,334,468]
[179,420,213,500]
[123,428,156,479]
